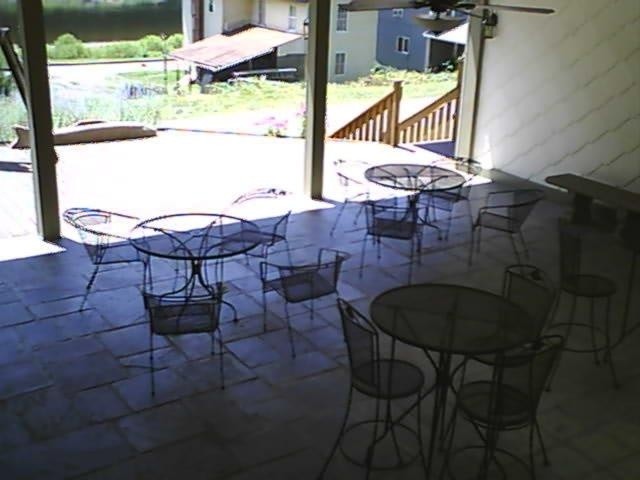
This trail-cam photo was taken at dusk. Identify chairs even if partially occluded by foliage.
[62,184,351,398]
[320,154,640,479]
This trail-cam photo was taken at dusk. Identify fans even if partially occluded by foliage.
[338,0,555,37]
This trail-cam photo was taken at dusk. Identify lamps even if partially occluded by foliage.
[412,10,467,37]
[480,8,498,40]
